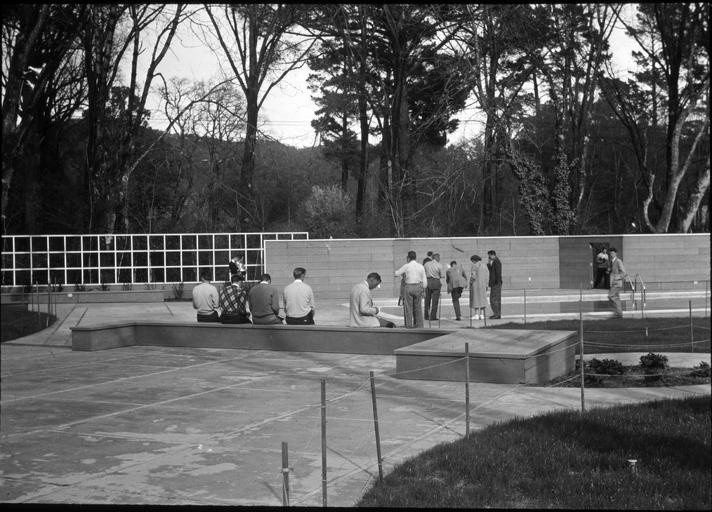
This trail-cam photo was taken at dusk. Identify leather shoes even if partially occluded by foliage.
[490,315,500,319]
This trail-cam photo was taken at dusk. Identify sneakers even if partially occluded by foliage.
[480,315,484,319]
[471,315,479,319]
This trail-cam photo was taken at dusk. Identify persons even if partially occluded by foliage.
[282,268,316,325]
[219,274,251,323]
[592,247,610,289]
[249,274,283,325]
[346,251,502,328]
[192,273,218,322]
[606,250,627,319]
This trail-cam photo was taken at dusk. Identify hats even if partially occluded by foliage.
[609,248,616,252]
